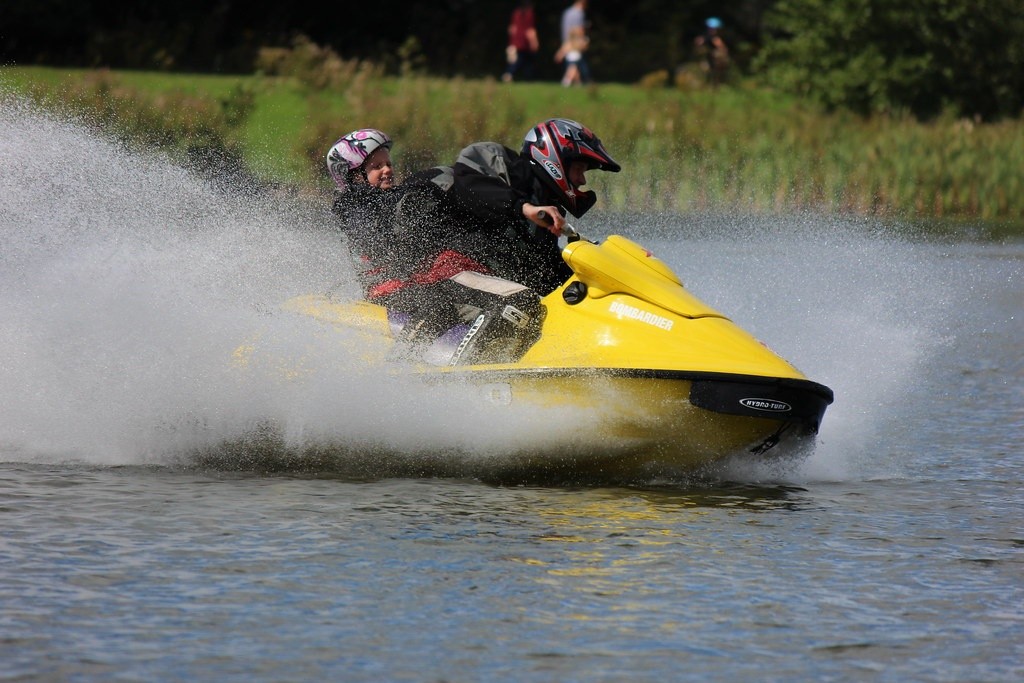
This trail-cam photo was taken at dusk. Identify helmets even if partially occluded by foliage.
[326,129,394,192]
[523,117,622,220]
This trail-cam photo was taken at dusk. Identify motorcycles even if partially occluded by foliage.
[230,209,834,479]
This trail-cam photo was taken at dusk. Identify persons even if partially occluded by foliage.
[693,18,729,82]
[438,119,621,381]
[508,0,539,81]
[326,130,459,370]
[561,0,590,82]
[555,24,588,87]
